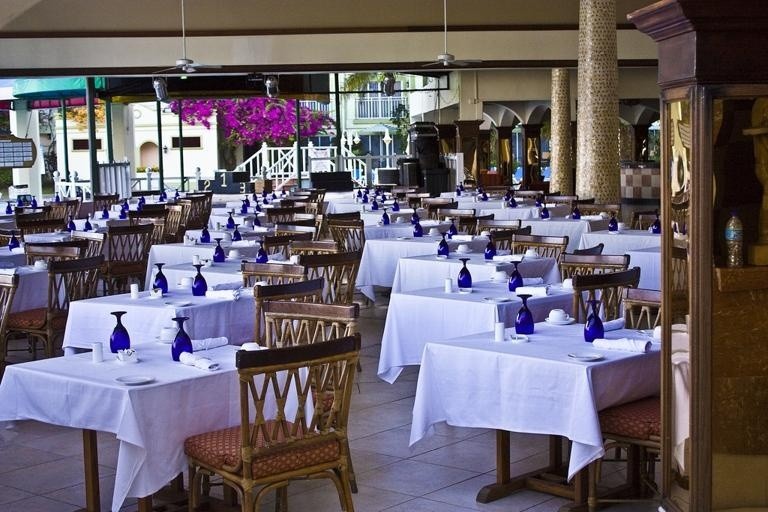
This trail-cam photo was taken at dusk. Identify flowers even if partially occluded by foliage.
[167,97,335,147]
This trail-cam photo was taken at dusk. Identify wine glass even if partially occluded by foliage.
[252,190,257,200]
[109,310,130,353]
[541,201,550,220]
[437,232,449,256]
[362,190,369,203]
[459,182,464,191]
[66,214,76,232]
[158,192,165,202]
[356,187,362,197]
[153,262,169,293]
[162,190,167,199]
[515,294,536,335]
[447,219,457,239]
[140,192,146,204]
[213,237,225,263]
[572,202,581,219]
[608,211,618,232]
[584,299,605,343]
[413,217,423,237]
[651,211,662,233]
[271,192,278,200]
[484,234,496,259]
[509,194,517,208]
[392,197,400,211]
[231,224,243,242]
[254,240,271,264]
[9,230,20,250]
[119,204,127,220]
[15,198,23,207]
[122,197,130,211]
[508,260,524,292]
[226,211,235,228]
[364,186,369,193]
[263,196,268,204]
[255,200,262,213]
[100,205,110,219]
[240,199,247,213]
[481,192,488,201]
[30,195,38,207]
[410,207,420,224]
[252,212,262,230]
[281,187,286,194]
[6,201,13,214]
[455,185,461,196]
[171,317,194,361]
[200,223,211,242]
[535,194,542,207]
[83,215,92,232]
[136,197,143,211]
[262,187,267,197]
[372,195,379,210]
[174,189,180,200]
[457,257,472,288]
[244,195,250,207]
[191,264,208,296]
[55,191,60,203]
[375,186,387,202]
[381,208,390,225]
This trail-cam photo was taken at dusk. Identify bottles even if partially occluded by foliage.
[724,210,744,270]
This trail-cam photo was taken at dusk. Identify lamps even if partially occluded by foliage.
[382,75,396,96]
[152,77,169,102]
[265,76,280,99]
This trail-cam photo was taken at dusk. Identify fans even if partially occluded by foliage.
[420,0,483,68]
[151,0,223,74]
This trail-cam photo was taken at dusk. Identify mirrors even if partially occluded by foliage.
[660,86,713,512]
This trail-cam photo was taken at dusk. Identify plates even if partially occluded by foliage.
[226,255,246,260]
[484,297,511,303]
[113,373,156,386]
[164,300,192,308]
[454,250,473,254]
[567,351,603,362]
[428,232,441,236]
[397,236,413,242]
[177,283,193,288]
[545,318,576,325]
[154,336,175,344]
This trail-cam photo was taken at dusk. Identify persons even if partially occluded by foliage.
[653,140,659,156]
[641,142,648,164]
[195,166,201,190]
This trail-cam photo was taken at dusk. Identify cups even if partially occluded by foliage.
[494,321,505,344]
[429,227,439,234]
[215,221,221,231]
[355,198,358,203]
[481,230,490,237]
[232,208,237,216]
[223,232,232,242]
[34,259,47,271]
[444,216,453,222]
[396,216,406,223]
[618,223,625,228]
[473,195,481,203]
[502,202,507,209]
[525,249,540,257]
[653,325,663,340]
[181,277,194,284]
[599,212,609,219]
[362,205,366,213]
[252,281,267,287]
[457,244,469,251]
[548,308,570,320]
[562,277,573,289]
[240,342,260,352]
[130,283,139,301]
[443,277,453,294]
[491,271,508,279]
[183,234,190,245]
[92,341,103,363]
[352,193,355,199]
[193,254,200,266]
[229,249,241,257]
[160,326,179,339]
